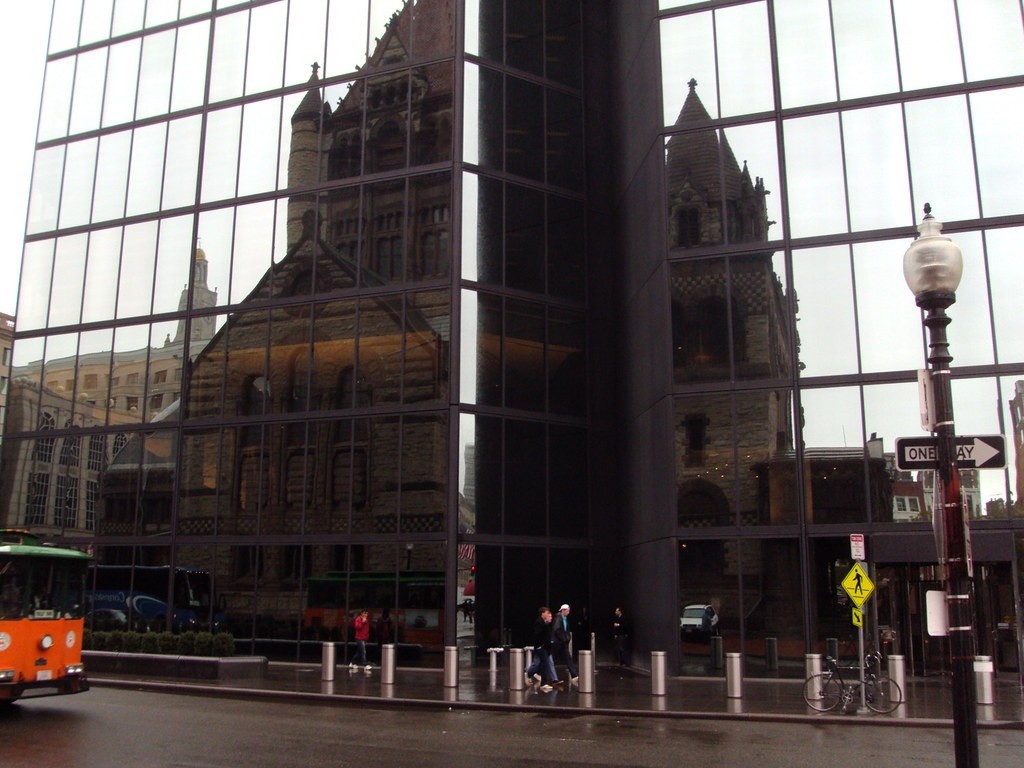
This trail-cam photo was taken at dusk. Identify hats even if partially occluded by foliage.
[559,604,569,611]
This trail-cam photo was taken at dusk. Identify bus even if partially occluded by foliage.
[78,563,229,634]
[0,528,93,705]
[302,572,447,657]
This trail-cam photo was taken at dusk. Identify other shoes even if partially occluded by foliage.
[349,663,358,668]
[525,673,530,686]
[366,665,372,669]
[540,685,553,688]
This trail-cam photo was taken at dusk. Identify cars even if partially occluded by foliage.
[680,604,719,640]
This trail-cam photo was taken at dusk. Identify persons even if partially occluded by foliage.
[463,600,473,624]
[0,576,49,616]
[524,604,578,690]
[341,610,427,644]
[349,608,372,670]
[613,607,628,667]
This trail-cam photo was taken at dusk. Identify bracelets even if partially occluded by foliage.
[617,623,620,627]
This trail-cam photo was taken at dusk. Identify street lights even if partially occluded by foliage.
[906,202,982,768]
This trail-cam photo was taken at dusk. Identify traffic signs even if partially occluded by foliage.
[897,434,1011,471]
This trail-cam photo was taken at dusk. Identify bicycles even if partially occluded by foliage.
[802,653,903,715]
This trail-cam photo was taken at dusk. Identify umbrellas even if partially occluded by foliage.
[463,598,473,602]
[346,609,357,616]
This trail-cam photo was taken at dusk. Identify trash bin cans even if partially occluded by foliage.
[464,645,477,667]
